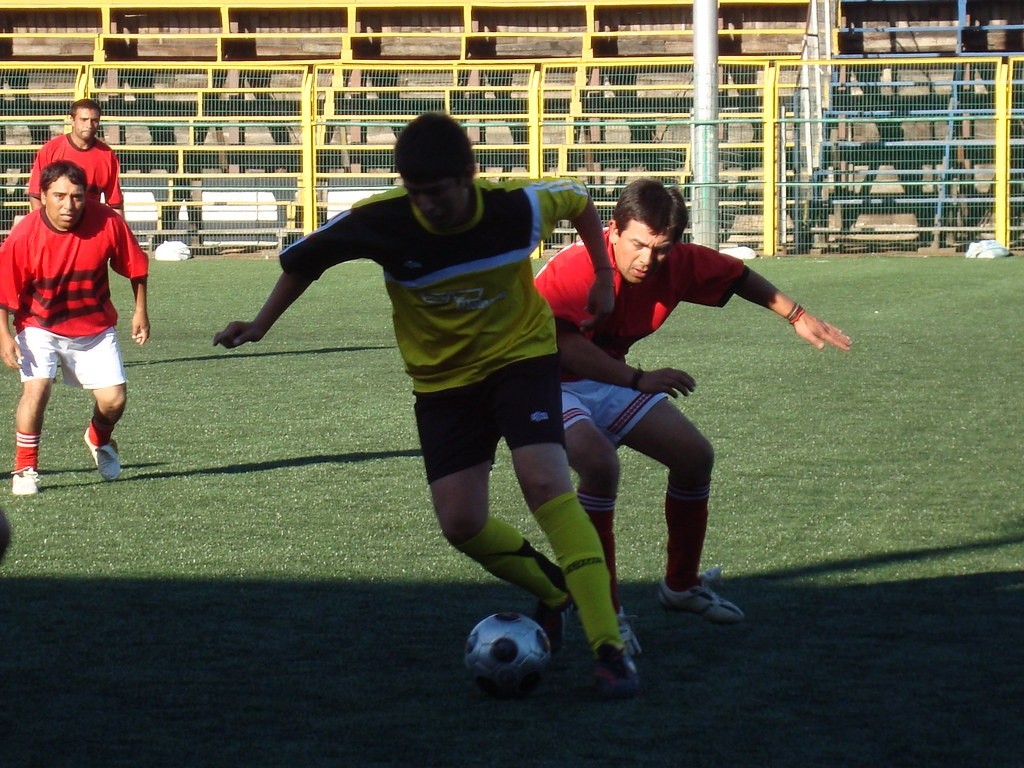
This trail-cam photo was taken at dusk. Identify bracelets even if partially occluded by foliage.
[593,266,614,272]
[785,301,806,325]
[631,367,643,389]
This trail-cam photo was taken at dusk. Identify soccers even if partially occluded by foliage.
[463,609,553,688]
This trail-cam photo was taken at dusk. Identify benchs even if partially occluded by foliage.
[0,0,1024,250]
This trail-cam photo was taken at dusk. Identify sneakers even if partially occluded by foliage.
[10,466,40,497]
[656,574,745,624]
[85,427,122,482]
[613,604,642,655]
[595,644,637,699]
[531,591,575,656]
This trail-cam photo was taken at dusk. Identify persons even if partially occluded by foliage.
[0,159,150,496]
[27,99,124,222]
[535,179,853,661]
[214,112,641,702]
